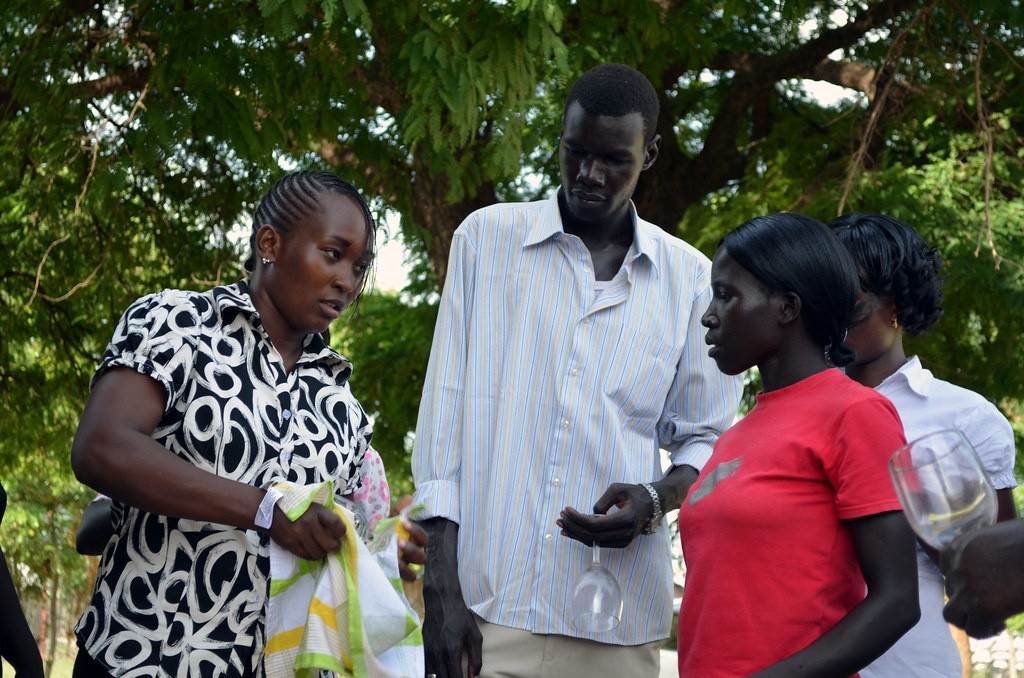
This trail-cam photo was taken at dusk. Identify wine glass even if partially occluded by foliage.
[570,538,624,633]
[888,427,998,551]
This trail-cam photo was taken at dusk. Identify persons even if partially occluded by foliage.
[939,517,1024,641]
[678,212,922,678]
[0,483,45,678]
[410,63,745,678]
[825,212,1018,678]
[71,168,428,678]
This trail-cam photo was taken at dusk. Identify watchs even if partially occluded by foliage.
[638,483,663,536]
